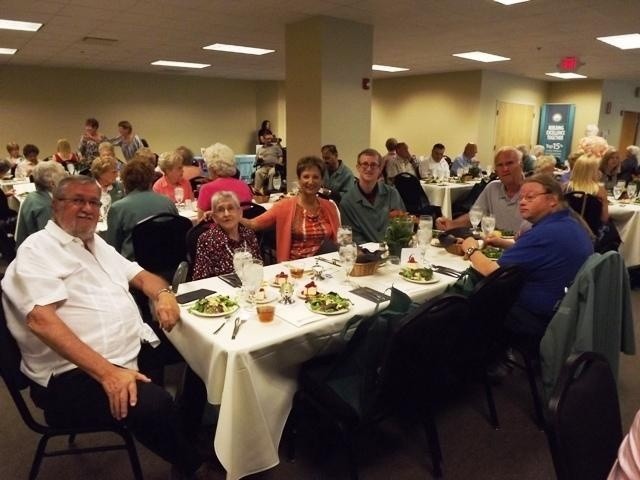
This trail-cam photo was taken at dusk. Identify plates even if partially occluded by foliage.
[399,273,440,284]
[187,300,240,317]
[305,300,354,315]
[269,278,290,289]
[262,286,279,304]
[483,246,500,261]
[502,234,514,239]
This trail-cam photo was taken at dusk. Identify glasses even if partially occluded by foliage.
[518,191,551,202]
[58,197,103,209]
[360,162,381,169]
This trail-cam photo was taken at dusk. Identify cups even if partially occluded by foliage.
[255,305,275,322]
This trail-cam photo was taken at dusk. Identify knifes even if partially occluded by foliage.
[214,312,247,342]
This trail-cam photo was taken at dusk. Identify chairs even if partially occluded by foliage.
[495,250,625,429]
[546,350,623,478]
[2,162,367,314]
[376,159,640,283]
[447,264,545,431]
[1,315,145,478]
[288,296,472,477]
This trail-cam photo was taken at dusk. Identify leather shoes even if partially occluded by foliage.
[191,462,227,478]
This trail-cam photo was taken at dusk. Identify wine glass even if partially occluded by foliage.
[173,184,185,205]
[432,165,491,181]
[335,224,357,291]
[468,205,496,237]
[412,215,434,267]
[231,247,265,311]
[611,178,638,201]
[287,261,305,291]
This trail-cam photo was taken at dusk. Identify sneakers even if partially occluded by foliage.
[496,354,517,379]
